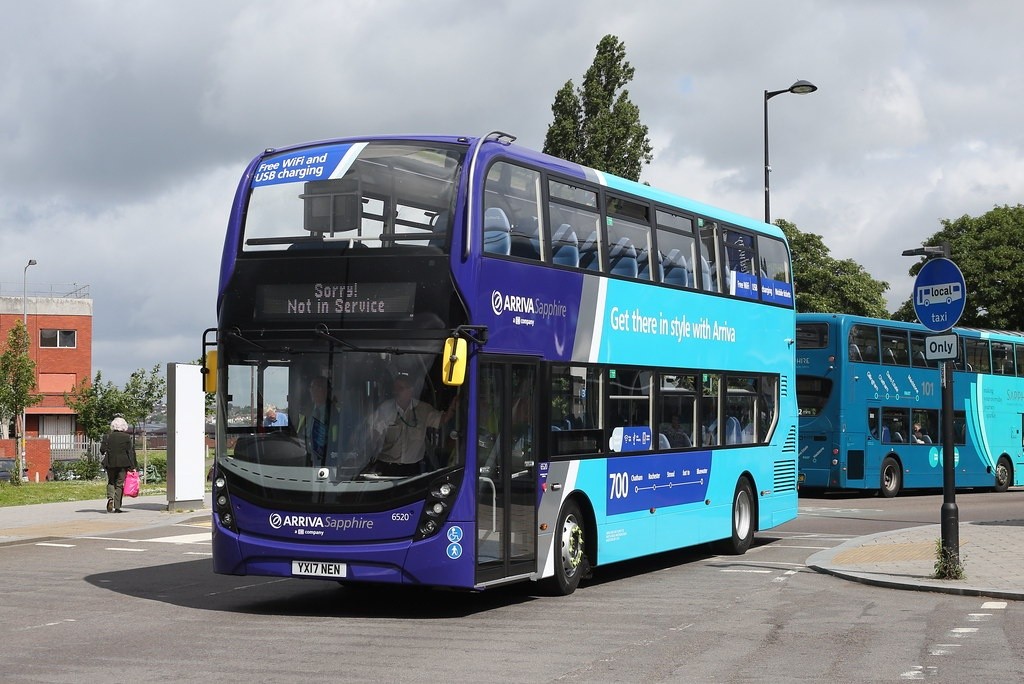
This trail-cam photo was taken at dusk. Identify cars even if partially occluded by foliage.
[0,457,29,482]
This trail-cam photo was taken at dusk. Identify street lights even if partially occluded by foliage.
[22,259,37,483]
[763,80,818,223]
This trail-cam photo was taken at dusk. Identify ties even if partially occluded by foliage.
[312,409,321,452]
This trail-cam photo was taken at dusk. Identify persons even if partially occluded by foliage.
[100,417,136,512]
[690,423,716,446]
[263,408,288,426]
[912,422,929,445]
[357,375,460,476]
[667,415,686,437]
[296,377,341,460]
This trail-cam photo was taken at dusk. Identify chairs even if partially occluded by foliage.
[908,434,917,443]
[848,341,974,371]
[550,414,756,453]
[923,435,933,444]
[894,432,904,443]
[428,207,713,295]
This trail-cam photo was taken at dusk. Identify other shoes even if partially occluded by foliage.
[115,509,122,512]
[107,499,113,512]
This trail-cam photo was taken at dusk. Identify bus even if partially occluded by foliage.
[201,131,799,597]
[796,313,1024,498]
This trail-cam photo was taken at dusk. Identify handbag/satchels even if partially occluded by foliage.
[123,469,140,498]
[102,434,110,469]
